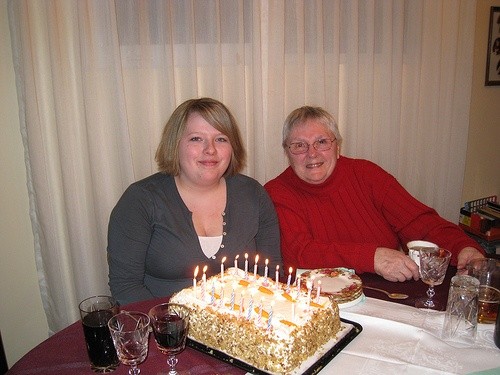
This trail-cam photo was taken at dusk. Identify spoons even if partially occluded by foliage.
[363,286,409,299]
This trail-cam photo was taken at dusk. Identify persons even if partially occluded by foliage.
[105,97,283,304]
[260,107,489,280]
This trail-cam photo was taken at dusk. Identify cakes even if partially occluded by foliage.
[168,266,340,375]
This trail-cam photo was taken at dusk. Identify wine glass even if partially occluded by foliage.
[416,247,452,311]
[108,311,151,375]
[148,303,190,375]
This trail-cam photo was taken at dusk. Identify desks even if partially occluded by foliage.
[4,264,500,375]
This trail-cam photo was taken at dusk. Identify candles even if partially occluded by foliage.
[193,253,322,331]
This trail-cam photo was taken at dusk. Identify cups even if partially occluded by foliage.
[442,274,480,348]
[466,257,500,325]
[406,241,439,278]
[78,296,120,374]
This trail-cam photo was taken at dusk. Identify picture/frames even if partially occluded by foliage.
[484,6,500,86]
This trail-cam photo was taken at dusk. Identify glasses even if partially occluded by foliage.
[287,137,336,154]
